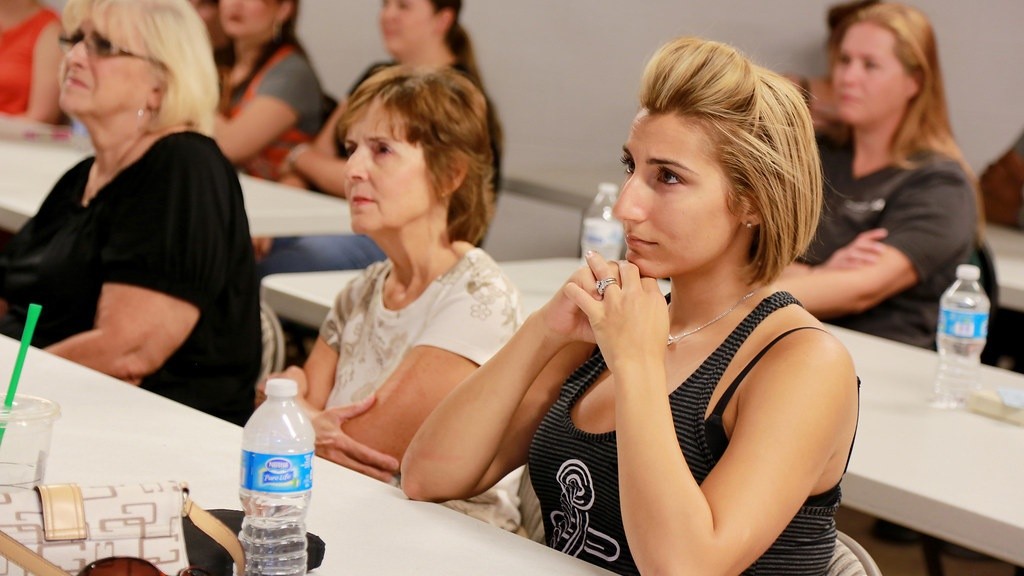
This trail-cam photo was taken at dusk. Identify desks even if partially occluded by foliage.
[986,222,1022,308]
[1,332,626,576]
[262,257,1024,568]
[0,139,368,235]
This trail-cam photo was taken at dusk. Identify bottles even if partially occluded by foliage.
[931,265,989,409]
[234,378,315,576]
[581,183,624,267]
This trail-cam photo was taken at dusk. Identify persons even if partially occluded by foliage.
[256,65,528,529]
[0,0,501,425]
[400,38,861,576]
[793,1,1024,545]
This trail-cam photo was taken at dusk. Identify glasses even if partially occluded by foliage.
[57,27,168,72]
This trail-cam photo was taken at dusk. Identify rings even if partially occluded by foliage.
[595,273,617,295]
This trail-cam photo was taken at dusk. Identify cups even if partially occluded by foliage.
[0,393,61,492]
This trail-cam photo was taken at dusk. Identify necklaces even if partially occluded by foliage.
[665,286,757,346]
